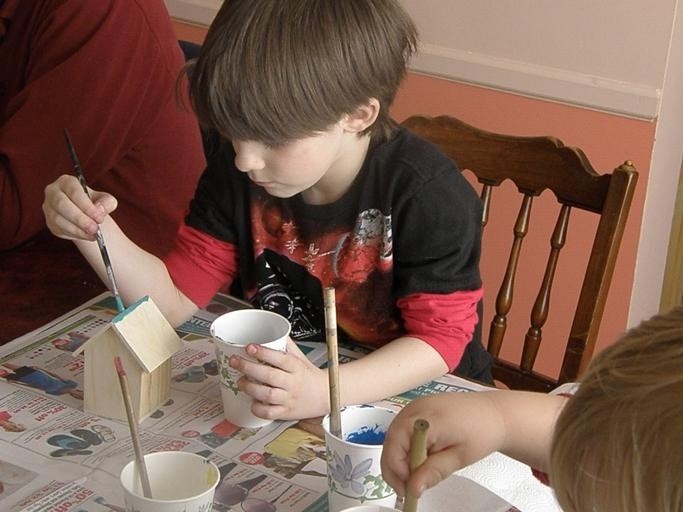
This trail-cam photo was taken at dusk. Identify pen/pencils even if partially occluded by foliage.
[115,357,152,498]
[403,419,429,512]
[323,286,342,438]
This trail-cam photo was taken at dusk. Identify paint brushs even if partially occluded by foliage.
[63,126,124,312]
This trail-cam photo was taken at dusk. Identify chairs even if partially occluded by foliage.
[179,39,225,165]
[396,113,638,393]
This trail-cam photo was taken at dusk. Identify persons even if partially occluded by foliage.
[0,1,209,352]
[377,303,683,512]
[37,0,496,424]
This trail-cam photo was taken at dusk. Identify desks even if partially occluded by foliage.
[0,229,502,512]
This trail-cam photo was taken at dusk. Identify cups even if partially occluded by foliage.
[321,404,402,512]
[209,309,291,428]
[119,450,220,512]
